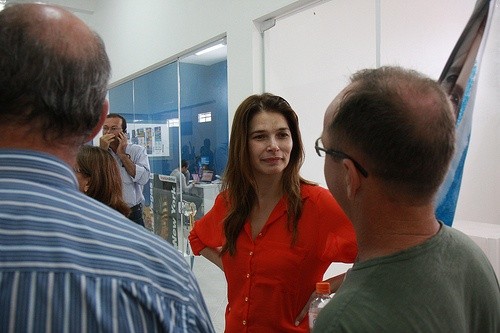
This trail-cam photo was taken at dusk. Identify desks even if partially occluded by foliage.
[189,179,219,217]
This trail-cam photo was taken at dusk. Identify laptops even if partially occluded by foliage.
[194,171,214,185]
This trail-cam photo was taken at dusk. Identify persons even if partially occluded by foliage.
[0,4,217,333]
[72,145,130,217]
[170,159,203,225]
[188,93,358,333]
[181,138,218,180]
[313,65,500,333]
[99,114,151,228]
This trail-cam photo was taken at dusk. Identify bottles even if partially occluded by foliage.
[308,282,333,333]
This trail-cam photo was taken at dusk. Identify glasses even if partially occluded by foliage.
[315,137,369,178]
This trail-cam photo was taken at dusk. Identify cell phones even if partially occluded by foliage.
[118,129,127,139]
